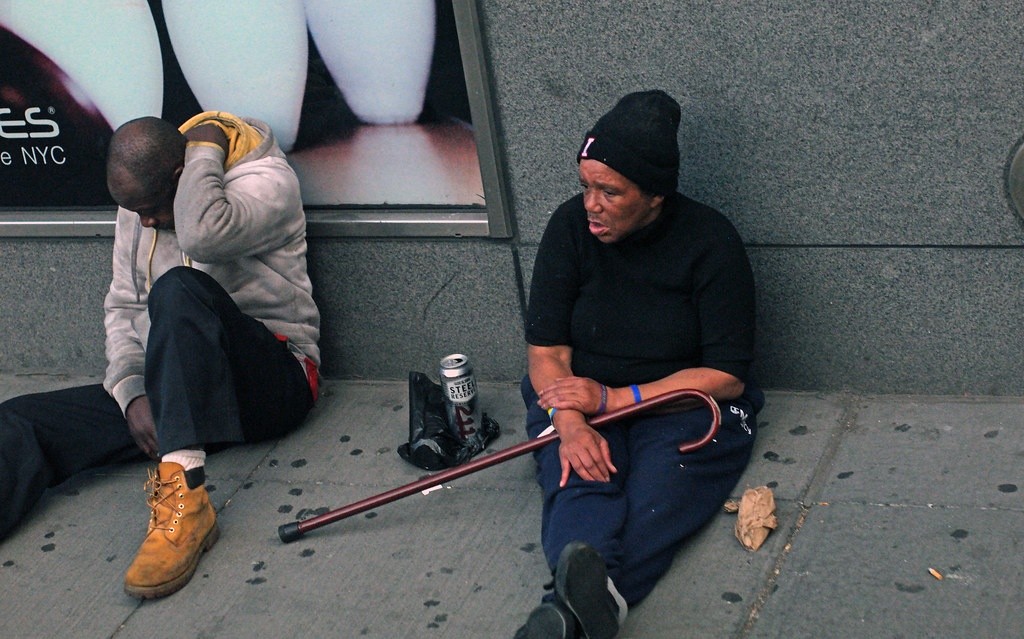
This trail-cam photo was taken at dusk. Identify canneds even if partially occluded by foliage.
[439,353,482,439]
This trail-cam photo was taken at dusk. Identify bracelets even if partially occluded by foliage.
[597,384,608,415]
[630,385,642,403]
[548,407,556,425]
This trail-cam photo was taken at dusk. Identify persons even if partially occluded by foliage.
[512,90,766,639]
[0,110,321,599]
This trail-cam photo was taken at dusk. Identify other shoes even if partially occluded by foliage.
[556,541,621,639]
[513,603,575,639]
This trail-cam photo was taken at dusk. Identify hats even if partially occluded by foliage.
[577,90,681,192]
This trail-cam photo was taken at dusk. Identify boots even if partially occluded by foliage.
[124,462,220,598]
